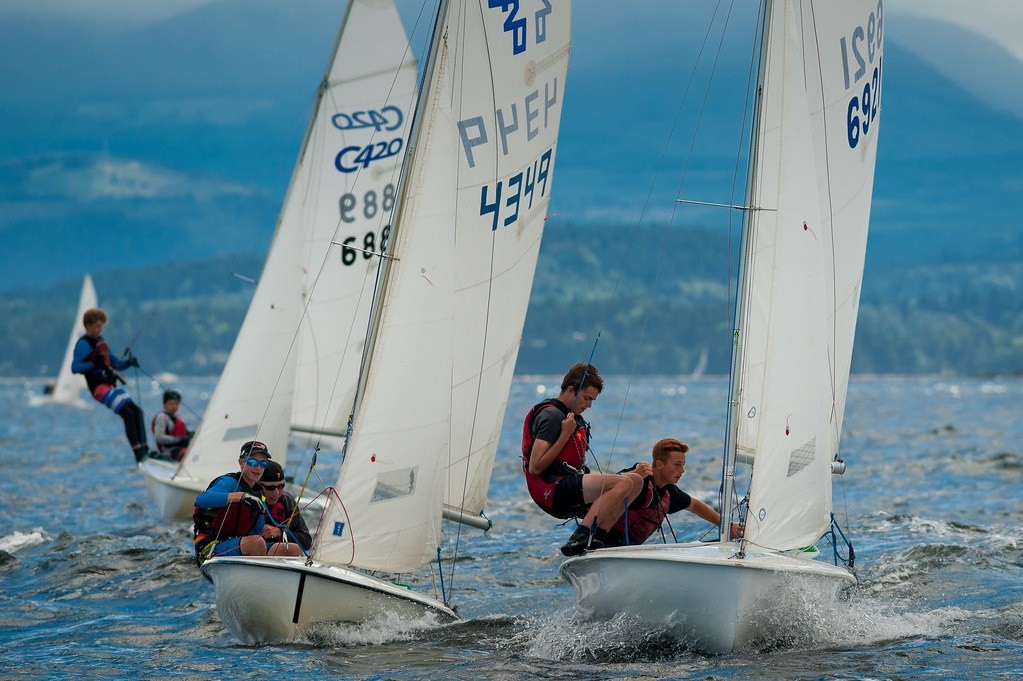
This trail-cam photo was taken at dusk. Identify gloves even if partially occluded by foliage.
[240,492,266,512]
[92,354,105,367]
[127,357,139,367]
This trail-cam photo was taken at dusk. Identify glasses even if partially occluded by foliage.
[243,457,270,469]
[259,481,285,492]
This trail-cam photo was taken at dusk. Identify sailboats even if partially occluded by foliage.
[21,270,99,413]
[198,0,577,650]
[555,0,889,659]
[137,0,419,538]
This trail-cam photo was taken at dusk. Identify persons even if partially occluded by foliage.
[191,441,303,568]
[608,438,745,547]
[257,460,313,551]
[71,309,157,461]
[520,363,644,557]
[43,380,55,394]
[151,389,195,461]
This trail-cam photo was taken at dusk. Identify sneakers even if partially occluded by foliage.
[560,534,604,557]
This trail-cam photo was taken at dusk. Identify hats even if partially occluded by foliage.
[259,460,284,482]
[239,440,272,460]
